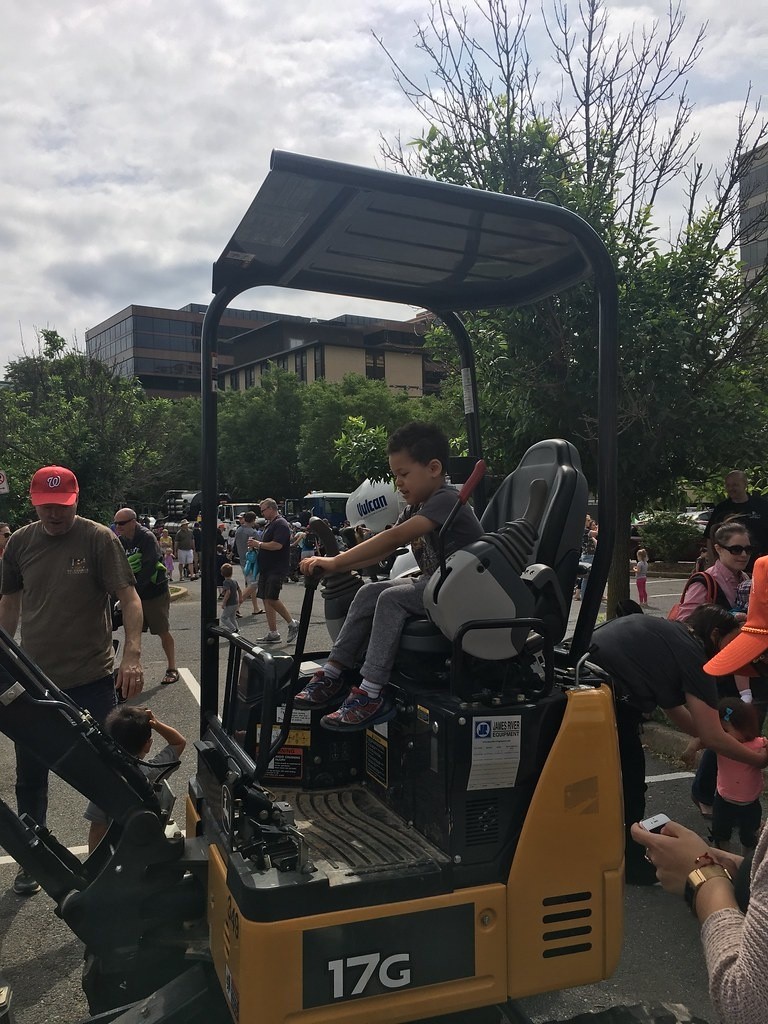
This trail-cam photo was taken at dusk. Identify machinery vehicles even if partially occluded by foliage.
[0,145,629,1024]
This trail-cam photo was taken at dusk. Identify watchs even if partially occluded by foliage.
[683,864,734,919]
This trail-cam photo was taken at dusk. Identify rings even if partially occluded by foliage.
[136,678,141,682]
[644,854,653,863]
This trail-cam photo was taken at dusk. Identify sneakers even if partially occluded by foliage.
[256,633,282,643]
[319,687,397,731]
[13,865,41,894]
[293,671,350,706]
[287,620,300,643]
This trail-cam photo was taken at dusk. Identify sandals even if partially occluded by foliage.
[161,669,180,684]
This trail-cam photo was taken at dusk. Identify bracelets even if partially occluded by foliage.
[688,851,738,879]
[260,542,262,549]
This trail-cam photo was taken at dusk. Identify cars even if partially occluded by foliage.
[634,511,713,534]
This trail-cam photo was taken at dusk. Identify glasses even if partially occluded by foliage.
[749,655,768,678]
[261,506,269,512]
[114,519,133,525]
[718,544,755,555]
[0,532,12,538]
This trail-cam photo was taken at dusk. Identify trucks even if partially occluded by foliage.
[218,501,261,543]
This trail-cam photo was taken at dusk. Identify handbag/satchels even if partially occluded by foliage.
[667,571,717,623]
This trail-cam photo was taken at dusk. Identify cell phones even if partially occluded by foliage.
[639,812,671,835]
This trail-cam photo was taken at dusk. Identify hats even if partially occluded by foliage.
[702,555,768,676]
[292,522,301,528]
[180,512,246,529]
[30,465,79,506]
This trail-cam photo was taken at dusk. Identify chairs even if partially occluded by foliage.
[395,437,590,701]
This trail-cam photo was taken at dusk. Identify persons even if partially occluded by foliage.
[0,522,13,601]
[292,419,486,733]
[109,498,393,686]
[544,470,768,886]
[630,553,768,1024]
[82,703,187,857]
[0,464,144,897]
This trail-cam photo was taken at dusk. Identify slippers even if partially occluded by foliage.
[235,611,242,618]
[252,609,266,614]
[574,595,581,601]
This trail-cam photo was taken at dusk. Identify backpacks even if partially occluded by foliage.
[302,532,316,548]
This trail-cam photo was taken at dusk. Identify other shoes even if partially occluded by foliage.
[167,573,199,582]
[690,793,712,819]
[234,628,241,633]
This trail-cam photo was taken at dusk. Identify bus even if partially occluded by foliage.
[303,490,348,526]
